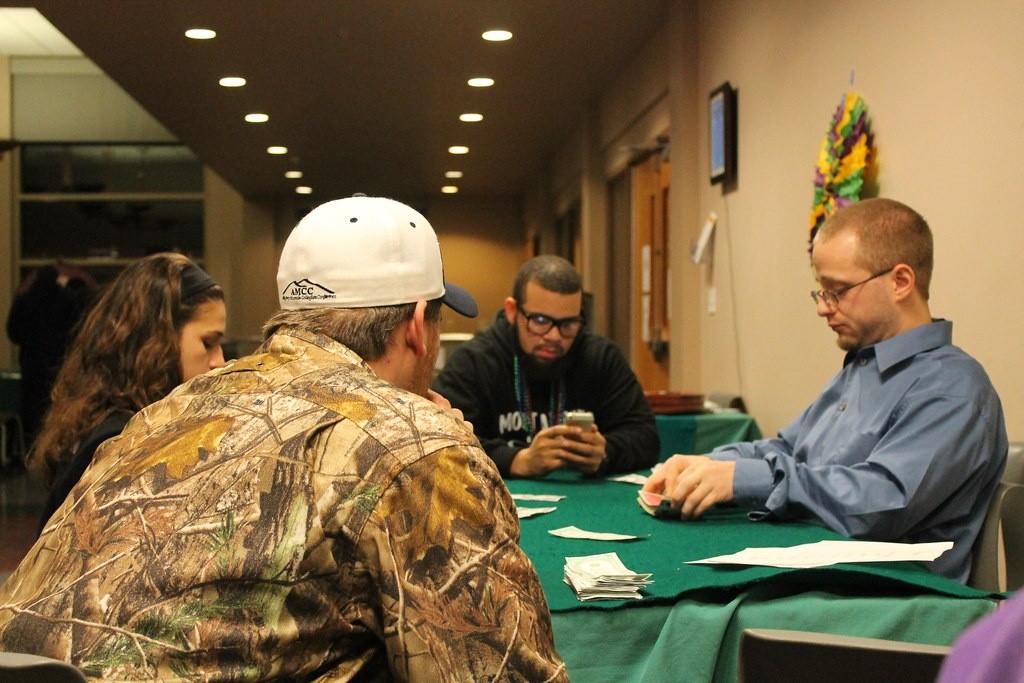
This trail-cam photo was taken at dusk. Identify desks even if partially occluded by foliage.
[644,396,761,460]
[500,457,1005,680]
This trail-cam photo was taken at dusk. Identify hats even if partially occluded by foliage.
[276,193,480,317]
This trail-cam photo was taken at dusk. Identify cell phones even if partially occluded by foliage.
[564,413,594,442]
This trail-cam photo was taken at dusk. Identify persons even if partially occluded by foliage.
[7,264,99,474]
[0,197,570,683]
[429,255,661,480]
[641,199,1008,586]
[25,252,226,544]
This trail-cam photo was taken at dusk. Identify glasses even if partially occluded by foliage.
[811,268,893,306]
[517,305,588,337]
